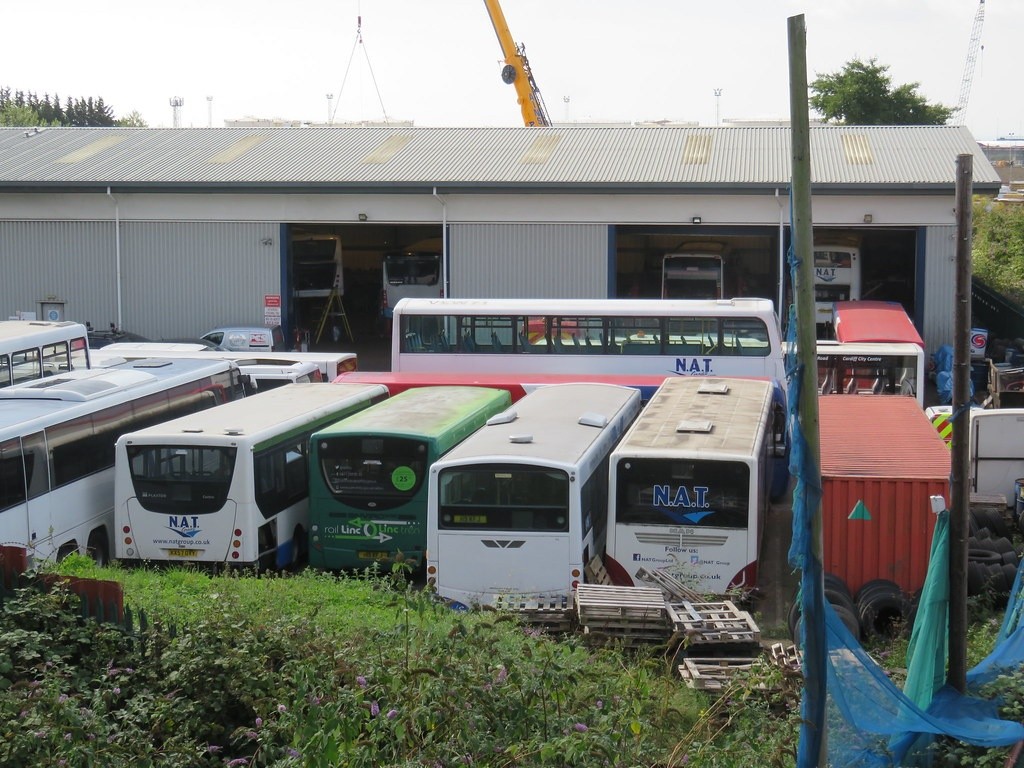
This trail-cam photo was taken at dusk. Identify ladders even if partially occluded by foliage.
[313,286,353,344]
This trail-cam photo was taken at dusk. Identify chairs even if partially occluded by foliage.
[820,366,916,395]
[406,333,745,356]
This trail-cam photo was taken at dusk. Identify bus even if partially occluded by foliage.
[0,317,92,392]
[114,384,392,573]
[380,252,444,320]
[423,382,644,629]
[219,352,361,384]
[811,233,863,341]
[291,232,346,298]
[657,240,733,300]
[533,334,927,413]
[236,356,323,398]
[0,356,260,583]
[390,296,793,400]
[308,387,513,579]
[829,298,924,385]
[605,373,777,606]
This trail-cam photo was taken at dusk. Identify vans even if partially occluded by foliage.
[102,324,288,365]
